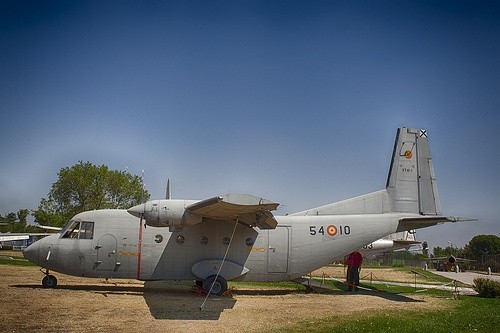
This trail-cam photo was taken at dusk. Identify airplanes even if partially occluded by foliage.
[21,125,481,296]
[424,253,478,266]
[360,228,422,252]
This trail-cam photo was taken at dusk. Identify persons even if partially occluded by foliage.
[344,251,363,292]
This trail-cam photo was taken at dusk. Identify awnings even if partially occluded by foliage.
[0,235,29,241]
